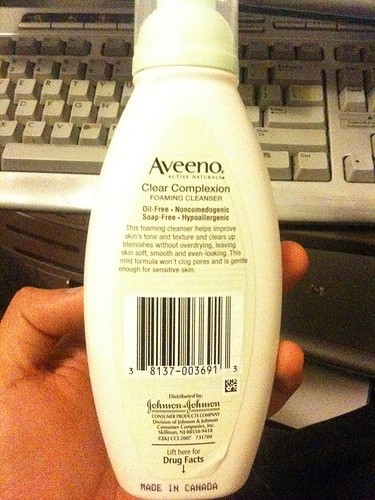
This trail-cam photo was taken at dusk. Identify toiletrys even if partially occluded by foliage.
[82,0,284,500]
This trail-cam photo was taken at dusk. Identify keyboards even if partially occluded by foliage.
[1,5,375,224]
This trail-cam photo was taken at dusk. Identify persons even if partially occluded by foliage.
[1,237,310,500]
